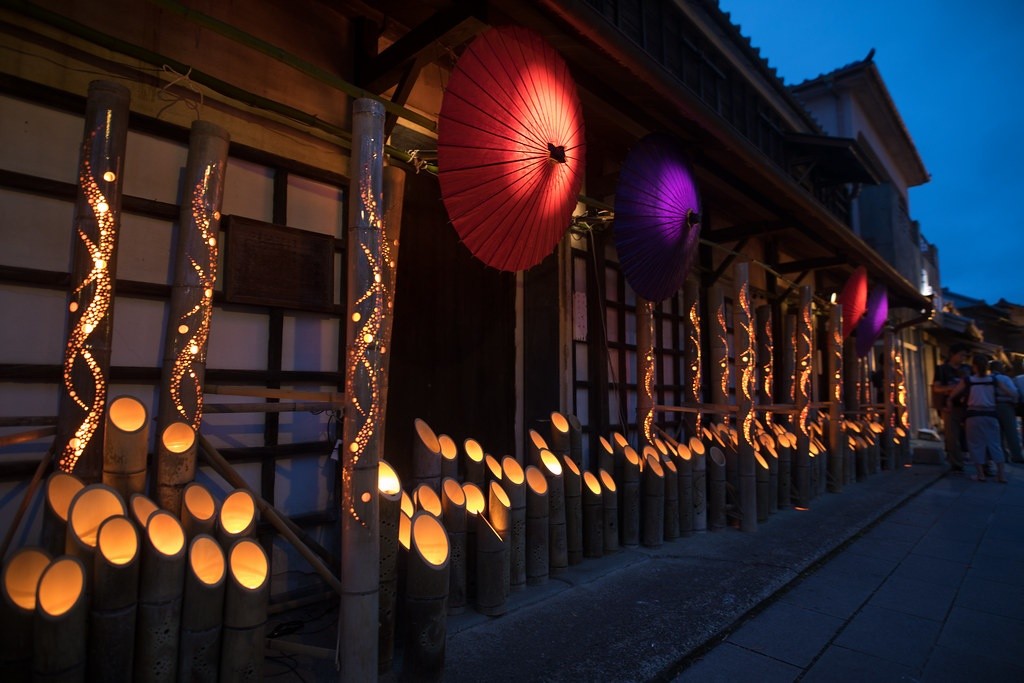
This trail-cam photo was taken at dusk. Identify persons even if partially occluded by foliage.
[932,344,1024,482]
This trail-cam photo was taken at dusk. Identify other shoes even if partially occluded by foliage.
[948,469,964,474]
[992,476,1008,483]
[1011,457,1024,463]
[976,477,986,482]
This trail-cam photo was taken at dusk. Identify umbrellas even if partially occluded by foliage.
[613,140,702,301]
[855,288,889,357]
[843,266,867,341]
[436,23,586,273]
